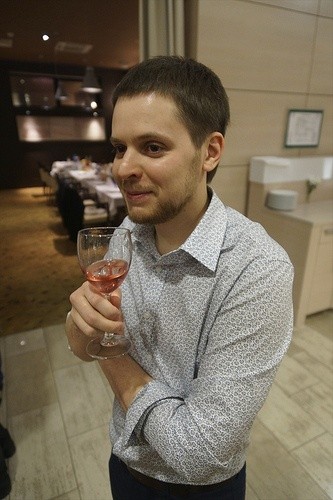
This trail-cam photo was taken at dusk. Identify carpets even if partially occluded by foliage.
[0,186,120,338]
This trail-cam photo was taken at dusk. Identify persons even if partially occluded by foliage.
[65,57,295,500]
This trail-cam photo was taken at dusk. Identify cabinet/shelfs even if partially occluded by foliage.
[246,157,333,323]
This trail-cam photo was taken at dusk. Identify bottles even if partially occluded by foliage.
[20,79,31,106]
[43,95,52,109]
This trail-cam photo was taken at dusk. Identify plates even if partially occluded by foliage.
[266,189,299,210]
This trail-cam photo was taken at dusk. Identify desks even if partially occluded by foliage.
[52,160,128,231]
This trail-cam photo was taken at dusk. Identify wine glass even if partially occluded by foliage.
[77,227,132,359]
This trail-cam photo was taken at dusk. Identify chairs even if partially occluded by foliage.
[40,167,110,240]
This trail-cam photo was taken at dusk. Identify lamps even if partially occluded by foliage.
[80,66,102,94]
